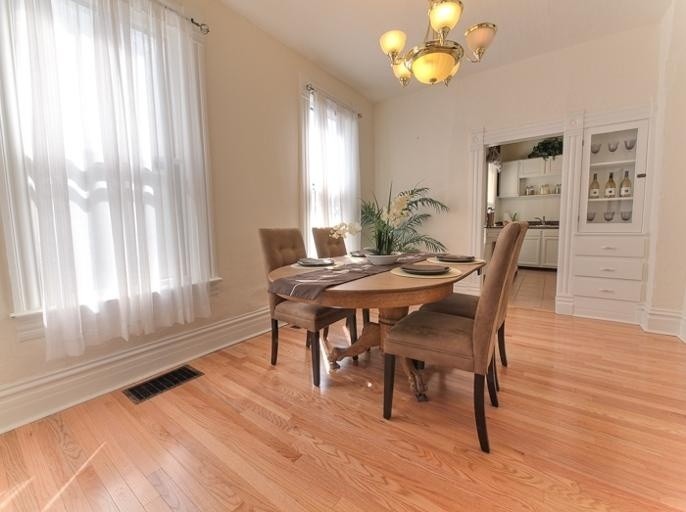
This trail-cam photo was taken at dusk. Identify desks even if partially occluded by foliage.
[269,244,486,401]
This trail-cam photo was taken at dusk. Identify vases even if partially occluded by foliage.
[361,249,403,267]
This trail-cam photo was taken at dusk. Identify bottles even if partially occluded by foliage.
[604,172,617,198]
[541,184,550,194]
[588,173,600,197]
[555,183,561,193]
[620,170,631,197]
[486,207,495,227]
[525,185,534,194]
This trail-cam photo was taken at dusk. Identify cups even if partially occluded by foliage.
[586,208,633,223]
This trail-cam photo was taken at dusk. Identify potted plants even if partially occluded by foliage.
[351,176,450,327]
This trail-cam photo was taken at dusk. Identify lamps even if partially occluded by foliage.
[378,1,496,90]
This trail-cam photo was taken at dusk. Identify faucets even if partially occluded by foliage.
[534,216,545,225]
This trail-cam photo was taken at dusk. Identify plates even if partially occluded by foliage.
[296,257,335,266]
[436,255,475,263]
[400,264,451,276]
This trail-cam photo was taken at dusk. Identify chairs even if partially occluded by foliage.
[255,228,360,389]
[379,221,522,455]
[419,221,530,367]
[311,225,372,355]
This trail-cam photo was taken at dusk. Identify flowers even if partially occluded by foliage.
[326,192,415,255]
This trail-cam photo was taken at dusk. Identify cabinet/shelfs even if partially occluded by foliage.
[497,160,525,197]
[569,231,645,325]
[576,116,649,234]
[518,228,558,270]
[518,154,561,178]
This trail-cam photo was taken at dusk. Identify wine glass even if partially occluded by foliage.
[591,137,636,164]
